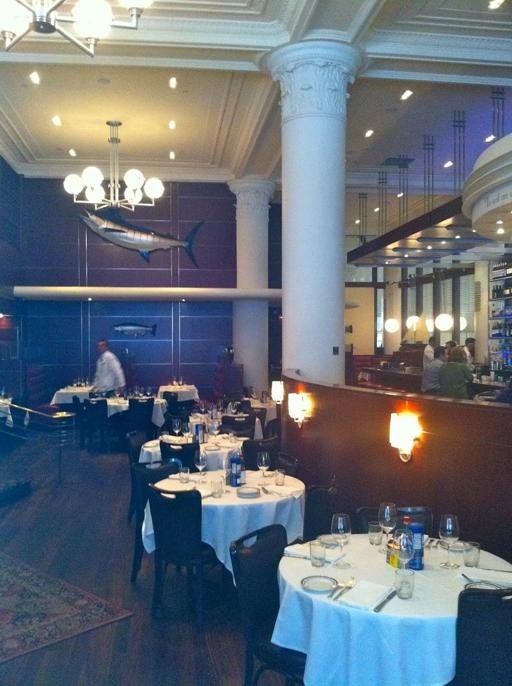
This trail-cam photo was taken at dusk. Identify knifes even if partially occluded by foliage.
[461,568,512,603]
[373,586,403,612]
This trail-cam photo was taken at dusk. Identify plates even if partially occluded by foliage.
[301,575,339,594]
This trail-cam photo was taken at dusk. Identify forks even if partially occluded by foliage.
[424,538,470,552]
[328,575,357,601]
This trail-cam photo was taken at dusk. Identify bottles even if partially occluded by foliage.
[487,257,512,354]
[167,374,185,388]
[385,515,424,571]
[223,343,235,363]
[69,375,90,391]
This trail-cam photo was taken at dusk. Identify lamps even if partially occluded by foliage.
[0,0,155,57]
[384,313,468,334]
[63,121,165,212]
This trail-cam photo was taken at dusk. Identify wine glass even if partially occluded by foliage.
[378,502,397,552]
[167,450,293,502]
[150,417,248,454]
[394,529,416,575]
[439,514,461,569]
[115,384,157,404]
[331,513,352,568]
[192,396,249,428]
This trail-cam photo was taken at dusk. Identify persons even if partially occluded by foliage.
[89,338,126,391]
[419,336,476,400]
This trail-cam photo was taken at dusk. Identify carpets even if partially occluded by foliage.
[0,550,136,665]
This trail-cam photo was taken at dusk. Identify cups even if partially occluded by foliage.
[464,542,480,568]
[368,520,383,546]
[395,569,414,598]
[473,354,512,387]
[310,540,325,567]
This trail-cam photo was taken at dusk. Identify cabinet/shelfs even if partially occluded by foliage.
[488,258,512,372]
